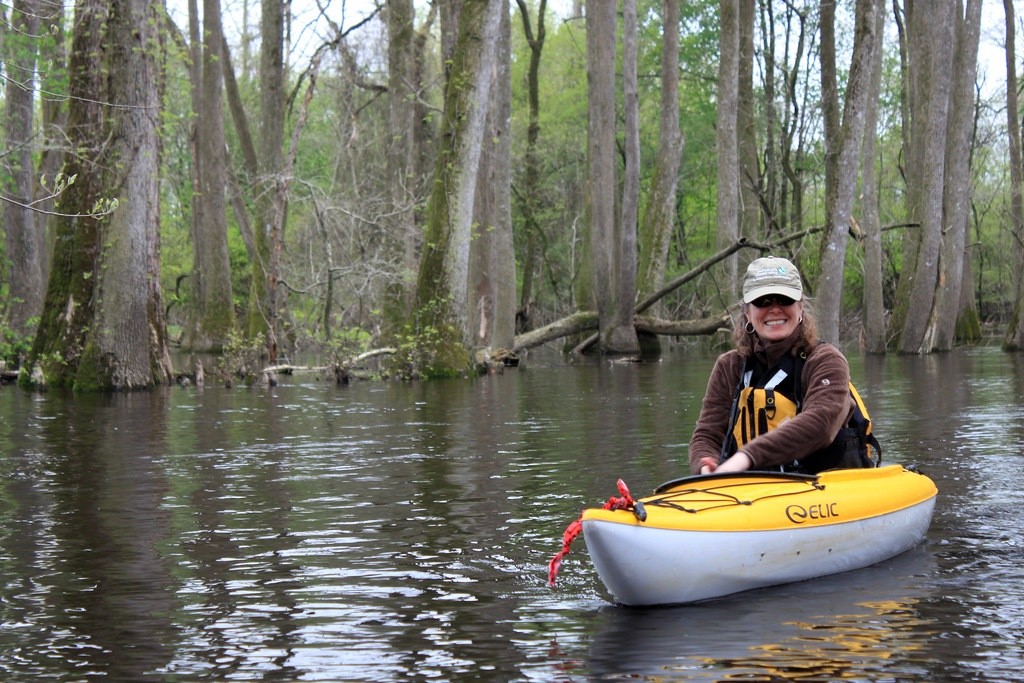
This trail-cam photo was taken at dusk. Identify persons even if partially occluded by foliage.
[689,256,859,478]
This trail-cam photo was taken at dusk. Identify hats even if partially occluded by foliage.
[743,256,803,305]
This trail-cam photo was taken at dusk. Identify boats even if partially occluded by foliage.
[575,462,941,612]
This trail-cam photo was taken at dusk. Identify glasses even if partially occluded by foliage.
[751,294,796,308]
